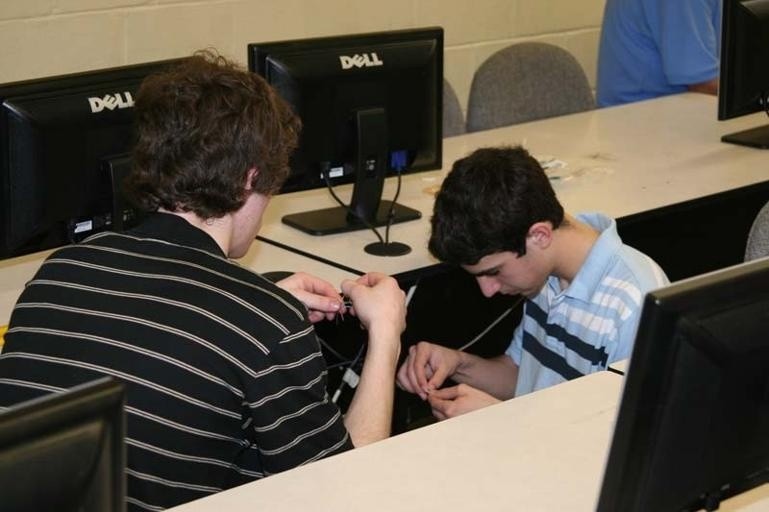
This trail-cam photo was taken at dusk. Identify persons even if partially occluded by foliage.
[396,143,673,421]
[0,47,407,512]
[596,0,723,108]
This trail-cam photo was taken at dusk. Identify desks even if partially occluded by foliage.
[254,90,768,290]
[138,370,768,512]
[0,233,365,325]
[606,357,632,376]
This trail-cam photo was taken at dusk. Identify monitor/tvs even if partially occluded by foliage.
[0,56,206,260]
[0,376,129,512]
[247,25,444,236]
[593,255,769,512]
[717,0,768,149]
